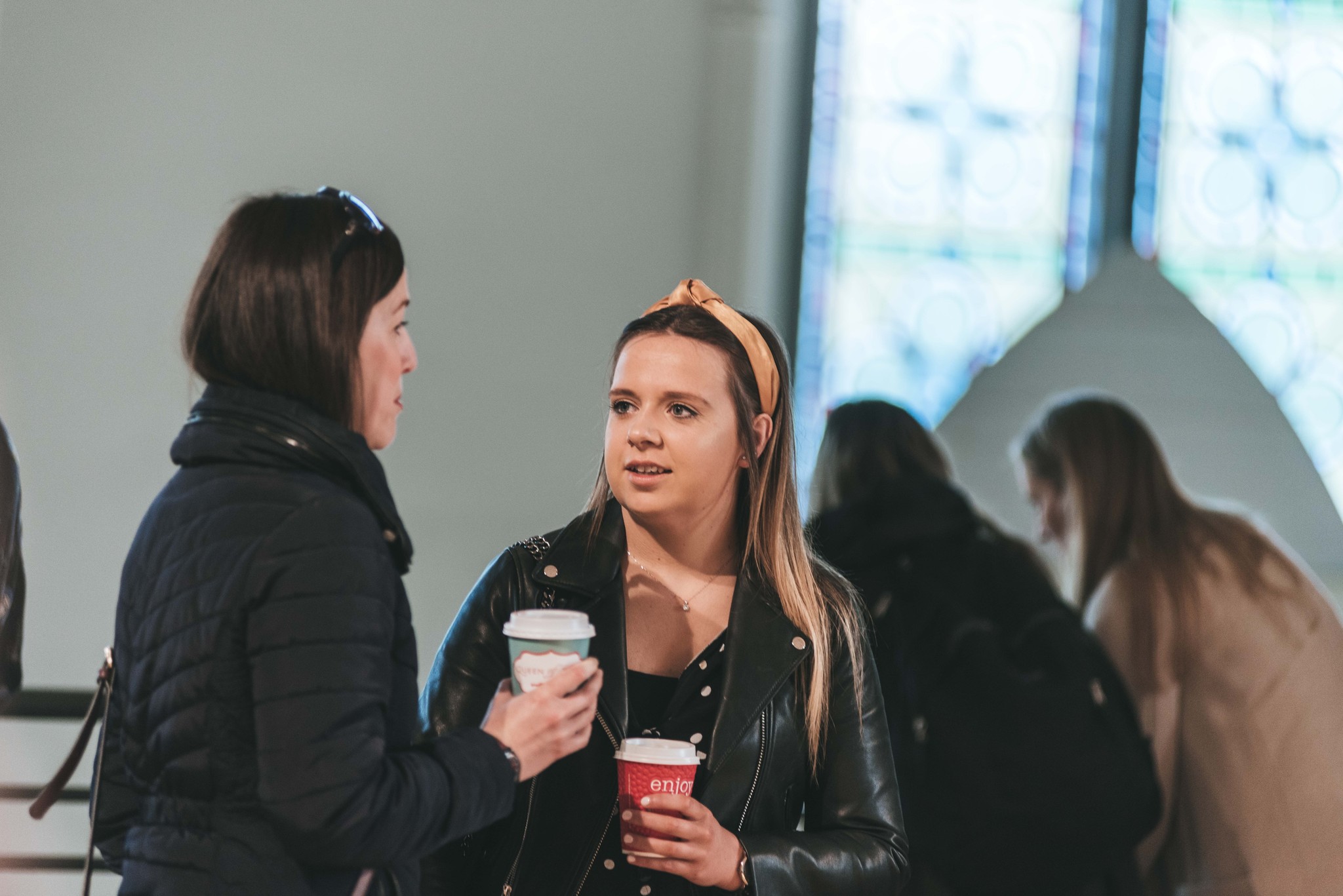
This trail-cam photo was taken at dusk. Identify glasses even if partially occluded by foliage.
[316,185,383,274]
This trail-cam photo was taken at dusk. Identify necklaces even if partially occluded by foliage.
[625,546,738,610]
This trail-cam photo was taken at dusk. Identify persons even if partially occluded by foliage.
[362,280,909,896]
[90,186,604,896]
[799,402,1166,896]
[1007,390,1343,896]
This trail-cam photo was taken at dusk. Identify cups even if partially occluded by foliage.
[503,610,595,698]
[614,738,700,860]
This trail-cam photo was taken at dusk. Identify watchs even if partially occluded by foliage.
[738,849,754,896]
[498,739,521,786]
